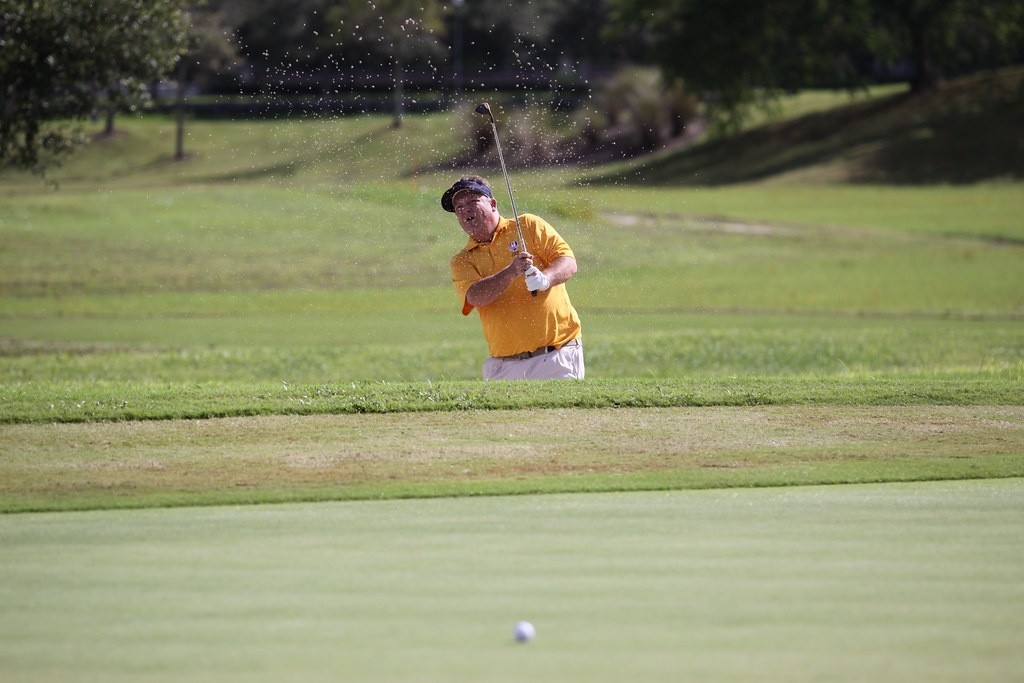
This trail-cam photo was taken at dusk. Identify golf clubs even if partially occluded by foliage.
[476,101,538,297]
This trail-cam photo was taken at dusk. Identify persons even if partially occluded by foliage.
[440,173,586,383]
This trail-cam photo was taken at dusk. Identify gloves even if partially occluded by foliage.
[524,265,551,292]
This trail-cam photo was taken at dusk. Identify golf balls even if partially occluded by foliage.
[515,620,535,644]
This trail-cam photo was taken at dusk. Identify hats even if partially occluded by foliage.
[440,181,493,213]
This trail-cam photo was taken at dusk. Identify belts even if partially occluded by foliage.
[498,346,557,361]
